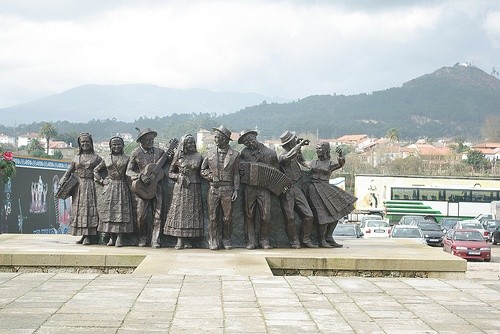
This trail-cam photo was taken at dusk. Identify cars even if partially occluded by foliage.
[332,213,393,240]
[409,219,445,247]
[442,229,491,263]
[397,215,425,226]
[388,223,428,245]
[440,213,500,244]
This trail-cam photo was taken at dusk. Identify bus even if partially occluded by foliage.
[382,180,500,230]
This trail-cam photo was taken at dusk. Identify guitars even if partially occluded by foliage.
[132,137,179,200]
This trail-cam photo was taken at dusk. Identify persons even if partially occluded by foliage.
[200,124,240,250]
[305,141,358,248]
[238,128,294,251]
[57,132,108,245]
[163,133,210,250]
[277,130,318,249]
[125,127,175,249]
[93,136,140,247]
